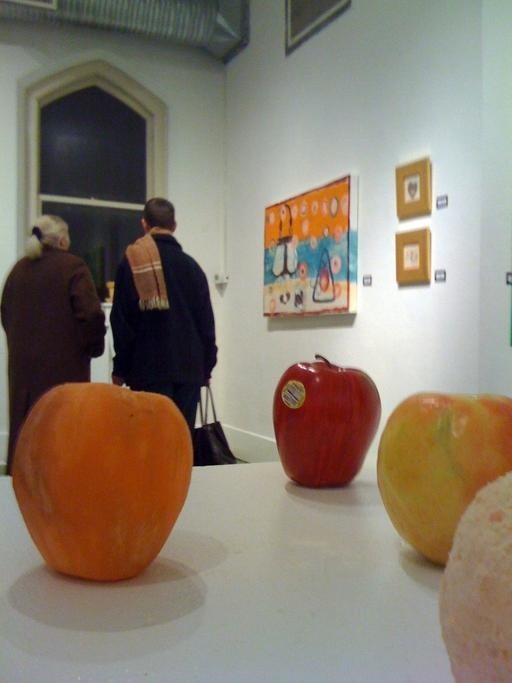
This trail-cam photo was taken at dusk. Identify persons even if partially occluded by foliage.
[0,216,107,476]
[110,196,217,430]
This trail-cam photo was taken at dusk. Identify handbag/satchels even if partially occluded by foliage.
[194,385,236,464]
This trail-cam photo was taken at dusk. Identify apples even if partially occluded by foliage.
[10,384,194,581]
[377,391,512,566]
[272,354,381,486]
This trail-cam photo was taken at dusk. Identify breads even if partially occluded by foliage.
[439,471,512,683]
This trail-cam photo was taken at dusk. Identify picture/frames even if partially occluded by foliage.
[395,156,432,223]
[282,2,350,54]
[395,228,433,287]
